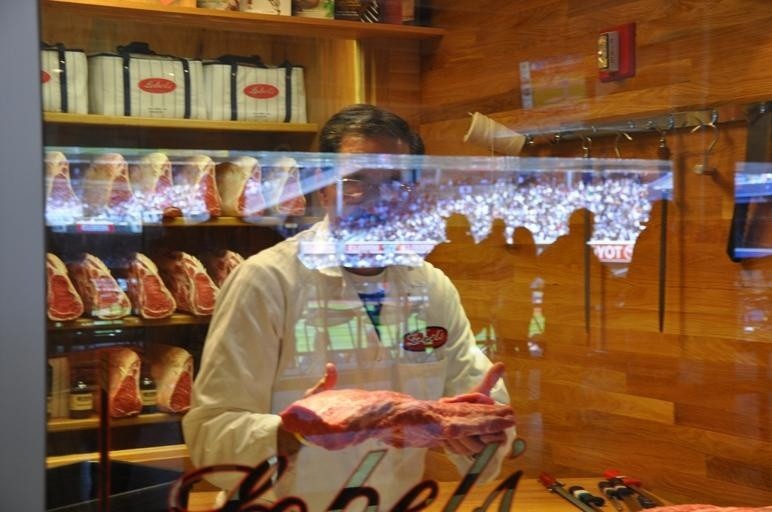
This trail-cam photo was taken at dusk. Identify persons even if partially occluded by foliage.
[179,101,520,509]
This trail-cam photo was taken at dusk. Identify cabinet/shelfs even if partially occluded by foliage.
[39,0,444,511]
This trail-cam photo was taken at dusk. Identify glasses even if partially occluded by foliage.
[331,175,411,200]
